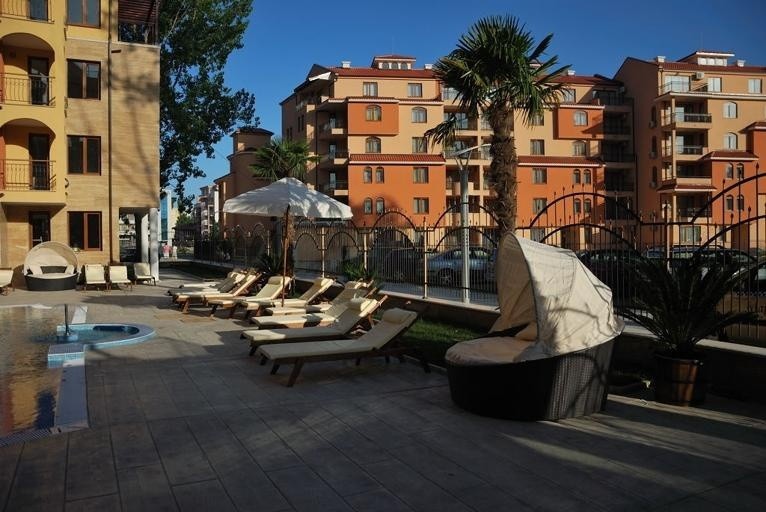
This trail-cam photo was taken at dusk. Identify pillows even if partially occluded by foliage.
[28,264,43,275]
[64,264,75,274]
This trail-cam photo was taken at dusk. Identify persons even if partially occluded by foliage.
[172,244,178,260]
[163,242,171,259]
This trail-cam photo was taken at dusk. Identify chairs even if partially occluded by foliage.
[84,262,156,291]
[167,263,432,389]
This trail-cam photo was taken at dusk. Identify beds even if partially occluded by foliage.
[22,239,80,292]
[446,231,626,422]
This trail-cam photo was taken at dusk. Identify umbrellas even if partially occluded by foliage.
[223,176,354,307]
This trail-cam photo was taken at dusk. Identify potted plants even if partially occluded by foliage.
[606,238,766,407]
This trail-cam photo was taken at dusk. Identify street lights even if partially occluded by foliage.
[446,143,491,303]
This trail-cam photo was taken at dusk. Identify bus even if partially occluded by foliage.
[648,244,766,280]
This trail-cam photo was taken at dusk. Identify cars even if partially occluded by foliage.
[423,247,492,285]
[341,253,386,274]
[384,248,438,283]
[574,251,657,294]
